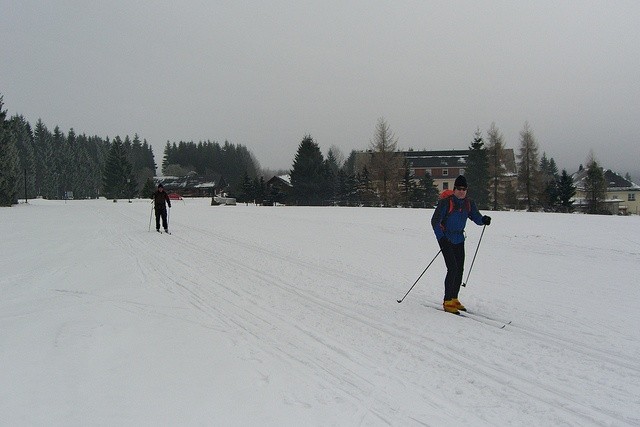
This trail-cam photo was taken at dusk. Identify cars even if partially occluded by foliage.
[167,192,183,200]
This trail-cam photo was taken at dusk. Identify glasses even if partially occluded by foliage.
[454,187,467,190]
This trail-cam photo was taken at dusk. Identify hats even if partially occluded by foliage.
[454,175,470,190]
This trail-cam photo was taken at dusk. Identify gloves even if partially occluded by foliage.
[483,215,491,225]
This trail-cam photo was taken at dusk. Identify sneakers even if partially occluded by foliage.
[452,299,464,309]
[444,301,457,312]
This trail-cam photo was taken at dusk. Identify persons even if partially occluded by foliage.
[151,183,171,233]
[431,175,491,314]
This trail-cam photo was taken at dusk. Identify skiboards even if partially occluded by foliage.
[157,230,172,235]
[422,297,512,329]
